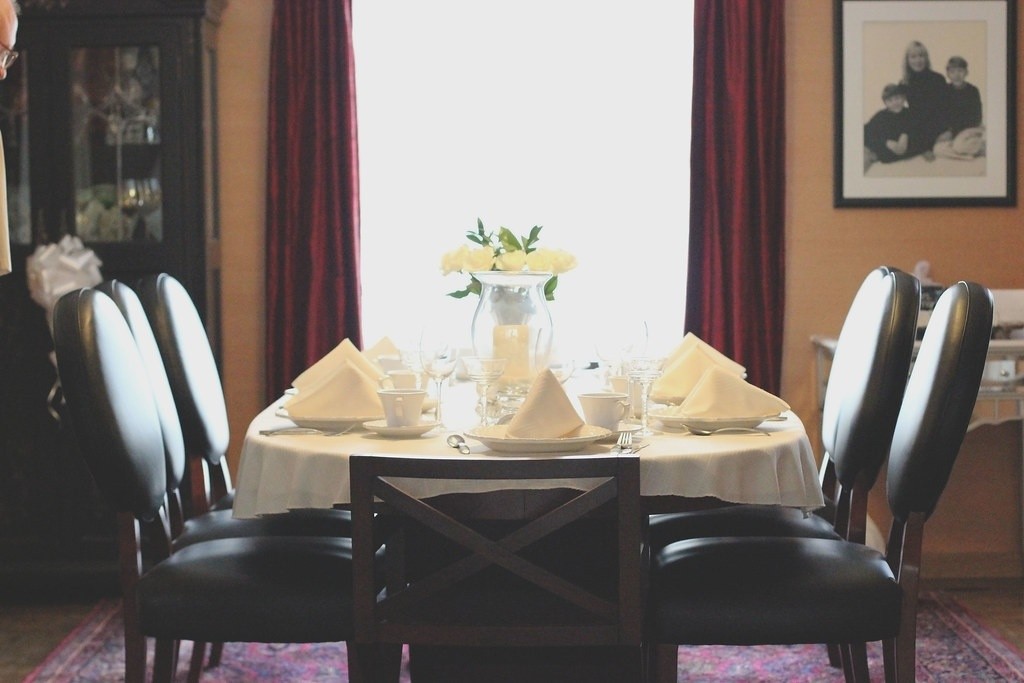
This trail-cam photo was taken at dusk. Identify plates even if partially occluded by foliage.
[647,393,780,432]
[598,424,643,441]
[276,388,441,439]
[464,425,613,452]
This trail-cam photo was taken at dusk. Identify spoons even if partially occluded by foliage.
[260,421,355,437]
[680,423,771,437]
[447,435,470,455]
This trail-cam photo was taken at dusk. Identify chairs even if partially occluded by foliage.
[129,270,351,514]
[52,287,387,683]
[349,456,647,683]
[643,265,994,683]
[94,278,351,683]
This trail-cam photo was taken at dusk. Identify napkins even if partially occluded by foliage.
[503,365,584,438]
[647,332,747,401]
[281,357,386,419]
[664,365,791,420]
[290,338,380,391]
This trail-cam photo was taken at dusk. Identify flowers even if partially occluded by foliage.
[434,216,580,302]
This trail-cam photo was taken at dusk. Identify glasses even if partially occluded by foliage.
[0,41,19,69]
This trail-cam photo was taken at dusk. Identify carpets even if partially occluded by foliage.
[20,578,1024,683]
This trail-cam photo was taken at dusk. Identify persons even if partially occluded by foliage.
[863,39,985,178]
[0,0,19,279]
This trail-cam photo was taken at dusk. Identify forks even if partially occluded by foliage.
[611,432,632,457]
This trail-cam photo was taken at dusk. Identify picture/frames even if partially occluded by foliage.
[829,0,1019,209]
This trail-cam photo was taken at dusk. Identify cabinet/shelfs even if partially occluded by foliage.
[812,331,1024,594]
[0,0,232,609]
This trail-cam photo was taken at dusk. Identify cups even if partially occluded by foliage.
[71,113,164,244]
[610,374,634,395]
[535,329,574,385]
[579,393,631,432]
[375,354,427,426]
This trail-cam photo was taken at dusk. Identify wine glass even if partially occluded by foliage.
[418,322,459,435]
[462,355,508,426]
[597,321,660,442]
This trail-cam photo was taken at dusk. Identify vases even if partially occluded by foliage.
[468,270,555,410]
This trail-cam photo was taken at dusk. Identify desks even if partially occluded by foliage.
[230,357,827,521]
